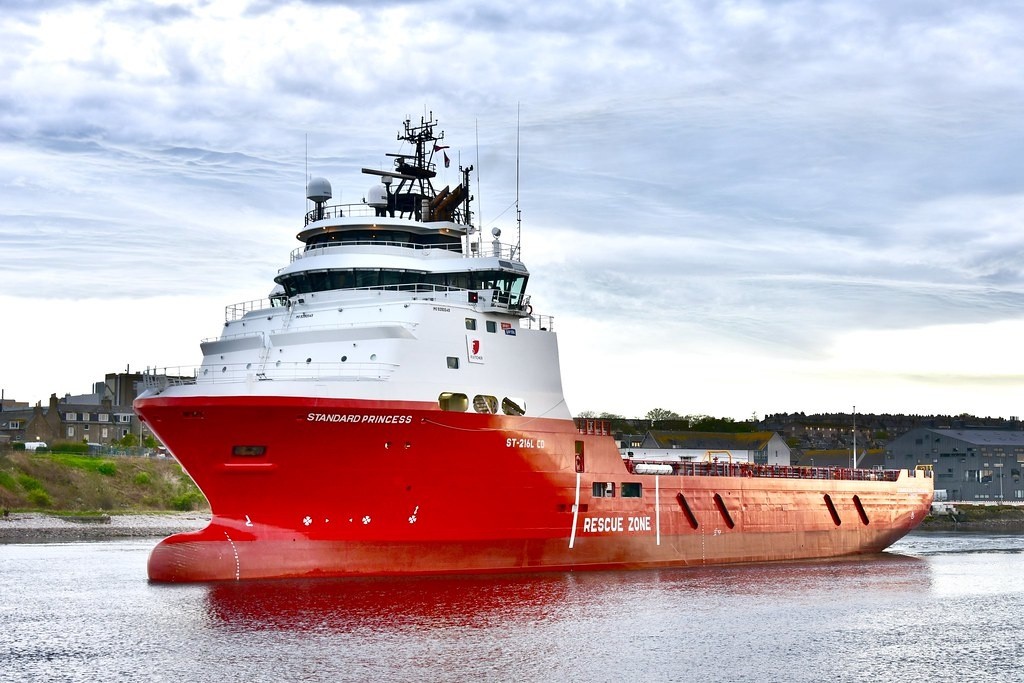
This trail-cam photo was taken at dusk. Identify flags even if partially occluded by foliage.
[444,151,450,168]
[434,145,450,152]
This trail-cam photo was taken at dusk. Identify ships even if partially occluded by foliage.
[132,104,937,584]
